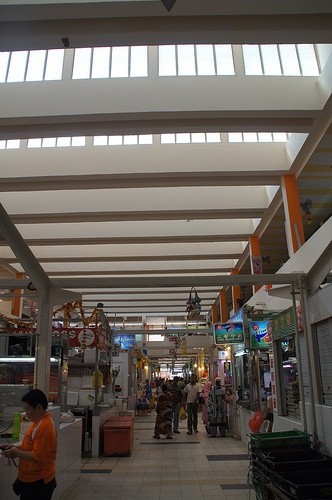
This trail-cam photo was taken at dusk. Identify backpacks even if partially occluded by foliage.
[197,391,210,405]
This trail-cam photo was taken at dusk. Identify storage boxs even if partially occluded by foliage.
[67,376,96,407]
[246,430,332,500]
[103,416,134,458]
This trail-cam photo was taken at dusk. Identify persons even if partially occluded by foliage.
[0,389,58,500]
[144,374,231,438]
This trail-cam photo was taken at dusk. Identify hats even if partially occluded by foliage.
[214,372,221,383]
[200,373,207,381]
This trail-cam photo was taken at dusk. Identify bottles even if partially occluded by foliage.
[12,415,21,439]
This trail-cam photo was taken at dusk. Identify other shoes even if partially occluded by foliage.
[205,426,208,433]
[166,436,173,439]
[173,430,180,433]
[195,429,198,433]
[187,432,192,435]
[208,435,215,438]
[153,435,160,439]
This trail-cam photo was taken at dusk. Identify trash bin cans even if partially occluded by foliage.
[248,429,332,500]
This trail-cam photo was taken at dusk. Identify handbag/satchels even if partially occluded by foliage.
[179,406,187,420]
[92,370,103,388]
[248,409,265,434]
[104,371,112,384]
